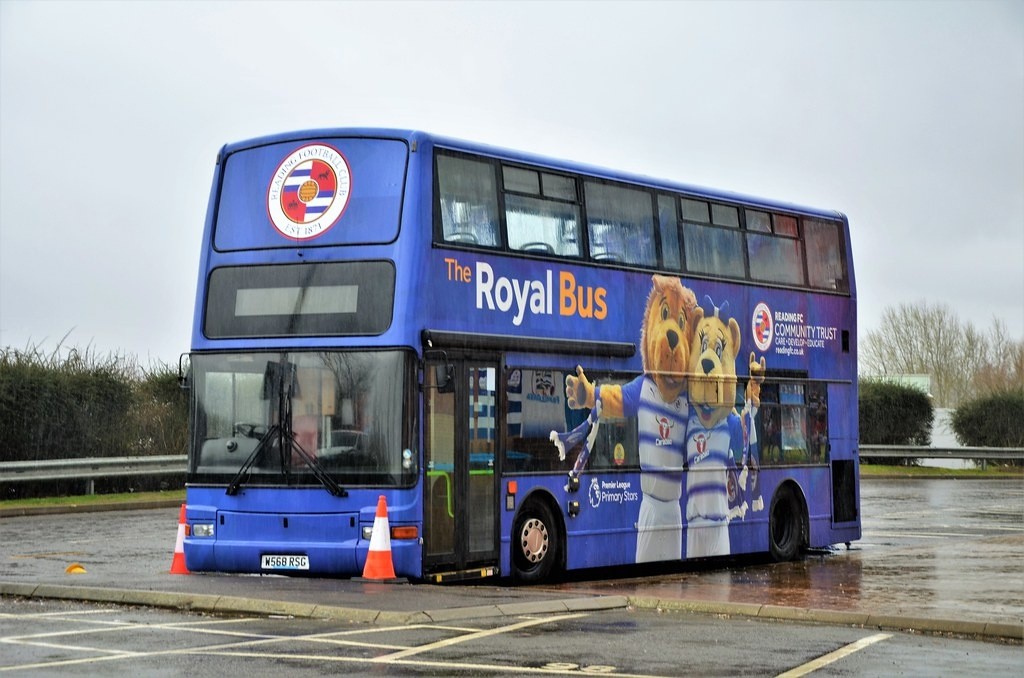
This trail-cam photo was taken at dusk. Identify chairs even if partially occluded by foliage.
[444,231,478,244]
[593,253,624,264]
[520,241,554,254]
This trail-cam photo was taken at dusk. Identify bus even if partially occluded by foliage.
[173,127,864,585]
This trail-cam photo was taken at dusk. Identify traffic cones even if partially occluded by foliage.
[348,493,411,585]
[168,503,194,575]
